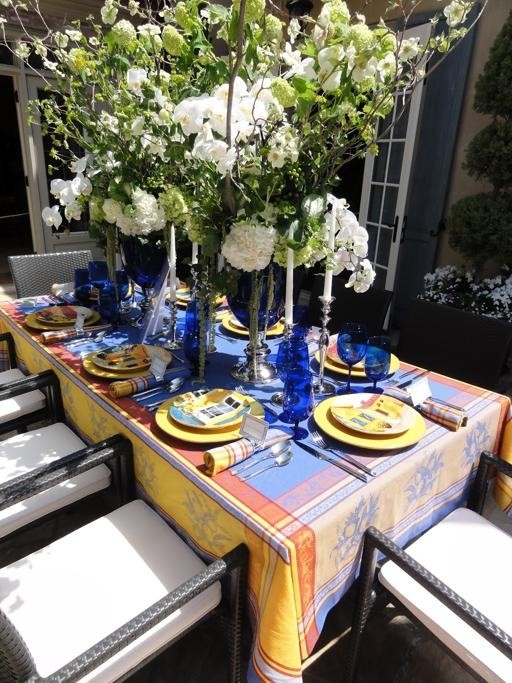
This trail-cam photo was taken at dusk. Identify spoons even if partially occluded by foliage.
[229,441,294,484]
[129,377,187,404]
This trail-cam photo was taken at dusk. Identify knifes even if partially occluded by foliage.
[387,366,432,389]
[294,439,368,486]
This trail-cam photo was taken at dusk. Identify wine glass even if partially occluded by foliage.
[185,301,213,378]
[74,260,131,327]
[337,323,389,396]
[276,339,314,440]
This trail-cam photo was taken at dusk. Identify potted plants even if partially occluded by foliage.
[418,12,512,321]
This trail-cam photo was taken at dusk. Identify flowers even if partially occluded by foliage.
[0,0,488,293]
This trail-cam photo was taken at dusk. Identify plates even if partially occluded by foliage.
[80,343,173,381]
[153,388,269,446]
[23,306,102,331]
[327,393,416,436]
[223,313,283,336]
[314,342,401,376]
[169,286,226,309]
[311,392,426,452]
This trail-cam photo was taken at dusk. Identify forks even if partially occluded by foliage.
[312,428,380,477]
